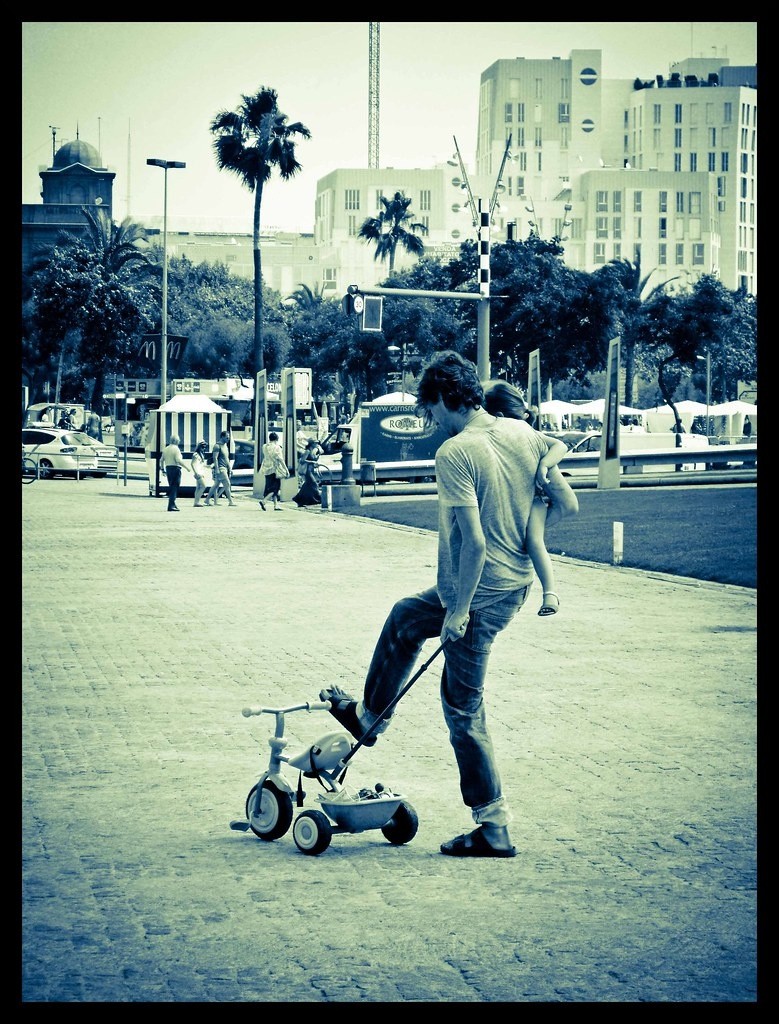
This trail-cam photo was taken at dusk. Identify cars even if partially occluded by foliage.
[22,428,119,479]
[232,439,255,468]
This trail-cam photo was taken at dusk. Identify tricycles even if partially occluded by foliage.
[229,699,420,855]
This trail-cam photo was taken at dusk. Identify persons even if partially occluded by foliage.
[191,439,213,507]
[481,380,568,616]
[58,411,71,430]
[204,431,237,506]
[743,418,751,437]
[597,422,602,432]
[627,419,634,426]
[258,432,290,511]
[585,421,593,431]
[292,437,322,507]
[160,434,191,511]
[68,409,77,430]
[673,419,686,433]
[319,350,580,857]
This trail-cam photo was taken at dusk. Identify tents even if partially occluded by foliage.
[538,398,757,445]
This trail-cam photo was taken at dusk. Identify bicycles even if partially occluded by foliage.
[21,446,38,485]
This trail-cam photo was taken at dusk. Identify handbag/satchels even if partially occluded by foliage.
[275,468,290,478]
[207,444,222,465]
[297,449,310,475]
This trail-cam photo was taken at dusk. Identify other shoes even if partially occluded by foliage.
[194,503,203,507]
[259,501,266,511]
[168,507,180,511]
[274,507,283,509]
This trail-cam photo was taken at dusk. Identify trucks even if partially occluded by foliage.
[556,432,709,477]
[298,402,452,482]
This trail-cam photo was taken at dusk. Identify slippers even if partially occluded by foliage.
[319,689,377,747]
[440,826,516,857]
[204,503,212,506]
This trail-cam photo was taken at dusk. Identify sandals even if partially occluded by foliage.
[537,592,561,615]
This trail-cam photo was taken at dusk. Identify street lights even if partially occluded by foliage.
[697,351,710,437]
[146,158,186,404]
[387,341,407,403]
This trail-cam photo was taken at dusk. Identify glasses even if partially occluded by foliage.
[201,446,206,449]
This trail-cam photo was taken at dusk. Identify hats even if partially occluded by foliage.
[307,437,319,443]
[198,440,208,445]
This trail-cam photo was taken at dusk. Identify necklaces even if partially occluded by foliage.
[465,411,487,425]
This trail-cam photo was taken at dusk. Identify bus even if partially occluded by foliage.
[102,393,171,450]
[209,395,337,476]
